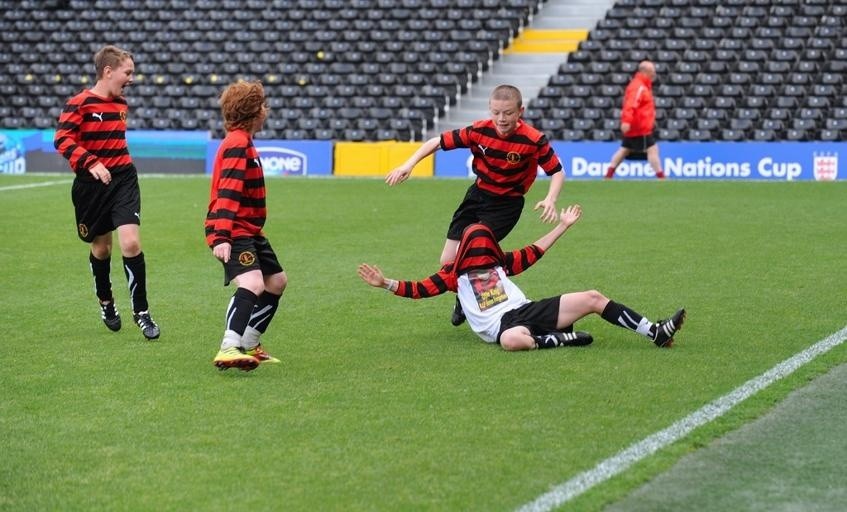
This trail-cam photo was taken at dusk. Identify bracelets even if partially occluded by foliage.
[388,280,395,291]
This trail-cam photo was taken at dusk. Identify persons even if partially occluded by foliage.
[384,84,565,325]
[204,80,288,370]
[603,59,666,179]
[357,203,685,351]
[52,45,162,340]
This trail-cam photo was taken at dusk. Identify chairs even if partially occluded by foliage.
[2,1,545,151]
[522,0,846,151]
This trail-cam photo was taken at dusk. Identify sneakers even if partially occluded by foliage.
[653,310,684,347]
[213,346,258,371]
[133,310,160,339]
[549,329,592,346]
[98,299,121,332]
[452,296,466,325]
[241,344,279,365]
